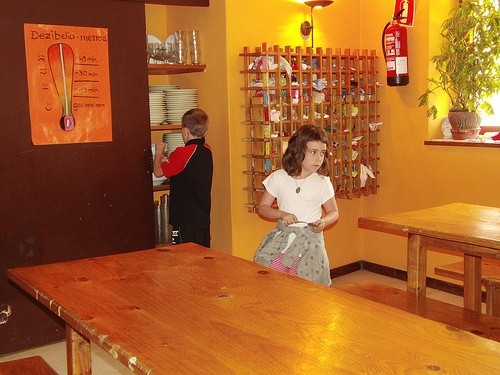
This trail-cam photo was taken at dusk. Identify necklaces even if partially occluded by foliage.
[295,172,311,193]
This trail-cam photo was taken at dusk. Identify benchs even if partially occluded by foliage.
[341,281,499,345]
[435,258,500,315]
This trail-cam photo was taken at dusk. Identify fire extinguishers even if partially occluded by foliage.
[381,9,409,87]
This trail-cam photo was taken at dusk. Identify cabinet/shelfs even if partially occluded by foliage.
[143,0,210,243]
[239,42,381,213]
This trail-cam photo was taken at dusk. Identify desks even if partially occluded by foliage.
[7,244,500,375]
[358,201,500,314]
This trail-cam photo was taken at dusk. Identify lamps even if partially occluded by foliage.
[301,0,334,50]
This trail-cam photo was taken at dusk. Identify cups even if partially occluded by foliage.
[146,28,200,66]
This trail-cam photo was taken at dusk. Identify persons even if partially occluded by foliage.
[252,122,340,286]
[152,107,213,248]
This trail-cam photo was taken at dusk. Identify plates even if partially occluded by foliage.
[148,85,198,188]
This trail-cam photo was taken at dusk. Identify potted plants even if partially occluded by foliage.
[413,0,500,141]
